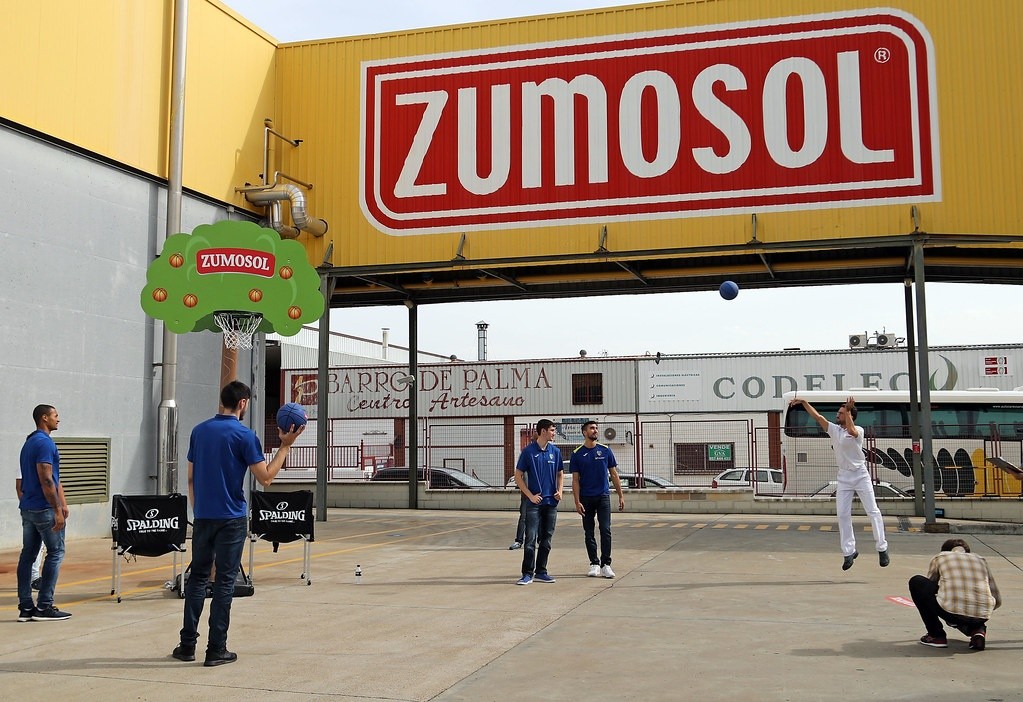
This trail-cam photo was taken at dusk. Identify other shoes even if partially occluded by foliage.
[842,549,859,571]
[919,634,949,648]
[967,633,986,651]
[878,550,891,567]
[509,541,522,550]
[204,648,240,667]
[172,642,196,662]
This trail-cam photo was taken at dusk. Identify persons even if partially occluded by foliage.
[513,419,565,585]
[14,404,73,622]
[569,421,625,578]
[508,432,539,550]
[907,539,1004,650]
[171,380,307,667]
[789,395,890,570]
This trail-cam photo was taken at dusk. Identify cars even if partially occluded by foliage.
[607,472,683,490]
[807,479,913,499]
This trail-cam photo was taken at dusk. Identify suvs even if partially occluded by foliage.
[370,465,501,489]
[506,459,581,493]
[711,465,785,498]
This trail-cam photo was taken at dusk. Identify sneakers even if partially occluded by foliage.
[31,606,72,622]
[602,563,616,578]
[533,572,556,583]
[516,574,534,586]
[16,607,38,622]
[587,564,602,577]
[30,577,45,592]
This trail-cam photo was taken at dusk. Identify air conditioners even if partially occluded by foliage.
[849,335,866,347]
[877,334,895,347]
[597,424,626,444]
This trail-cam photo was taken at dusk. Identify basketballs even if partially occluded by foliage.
[719,280,739,300]
[276,403,308,433]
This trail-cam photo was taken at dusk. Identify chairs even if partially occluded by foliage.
[111,492,187,601]
[249,489,314,586]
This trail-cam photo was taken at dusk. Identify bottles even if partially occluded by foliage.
[355,565,361,585]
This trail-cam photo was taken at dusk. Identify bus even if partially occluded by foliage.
[779,385,1023,498]
